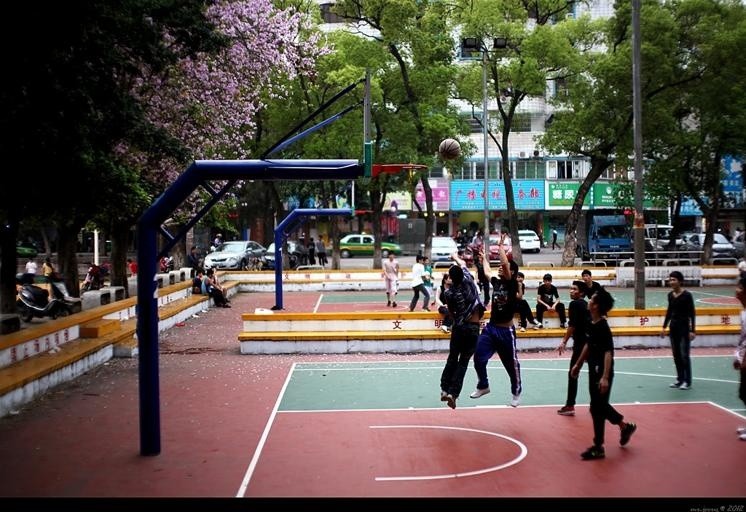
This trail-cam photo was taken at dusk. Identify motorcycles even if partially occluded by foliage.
[15,269,73,326]
[79,260,110,293]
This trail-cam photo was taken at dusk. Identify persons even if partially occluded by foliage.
[17,223,746,441]
[572,287,636,458]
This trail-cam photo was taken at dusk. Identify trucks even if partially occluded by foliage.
[575,209,632,259]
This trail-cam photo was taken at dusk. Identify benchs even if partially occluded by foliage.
[0,267,238,422]
[226,265,746,296]
[236,307,745,359]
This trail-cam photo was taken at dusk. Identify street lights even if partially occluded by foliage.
[459,35,510,264]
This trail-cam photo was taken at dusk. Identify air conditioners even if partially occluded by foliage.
[516,148,529,159]
[499,95,507,103]
[529,147,545,158]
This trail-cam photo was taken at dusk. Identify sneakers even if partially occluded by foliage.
[511,395,519,407]
[620,422,636,446]
[440,391,447,402]
[441,325,447,332]
[519,318,544,332]
[736,427,746,433]
[739,434,745,440]
[423,306,430,311]
[560,322,568,328]
[669,380,680,388]
[580,448,604,459]
[679,382,689,389]
[446,394,455,409]
[469,388,490,398]
[393,303,397,306]
[557,407,575,415]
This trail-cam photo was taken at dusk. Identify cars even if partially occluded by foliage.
[203,240,267,271]
[263,238,304,270]
[489,233,512,266]
[731,229,746,253]
[419,235,462,268]
[518,228,542,254]
[340,231,403,259]
[678,232,737,264]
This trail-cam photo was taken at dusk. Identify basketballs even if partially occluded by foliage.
[439,139,461,160]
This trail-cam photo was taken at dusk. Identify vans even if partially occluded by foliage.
[644,221,688,251]
